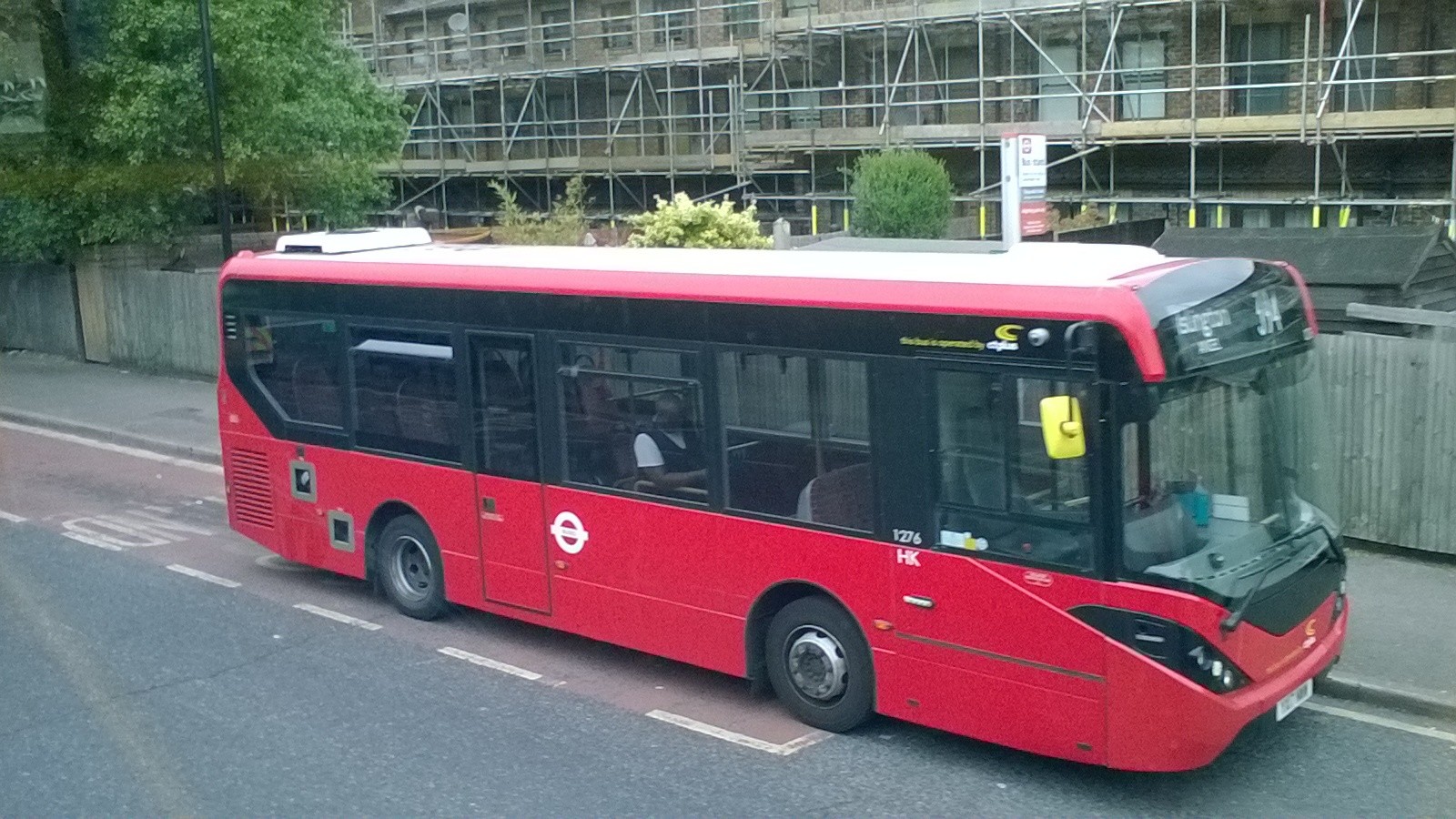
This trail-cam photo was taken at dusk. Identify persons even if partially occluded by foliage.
[634,394,709,501]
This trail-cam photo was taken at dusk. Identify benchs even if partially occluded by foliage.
[797,462,882,527]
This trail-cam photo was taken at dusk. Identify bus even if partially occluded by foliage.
[216,226,1350,772]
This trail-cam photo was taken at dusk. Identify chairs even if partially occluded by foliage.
[291,353,639,486]
